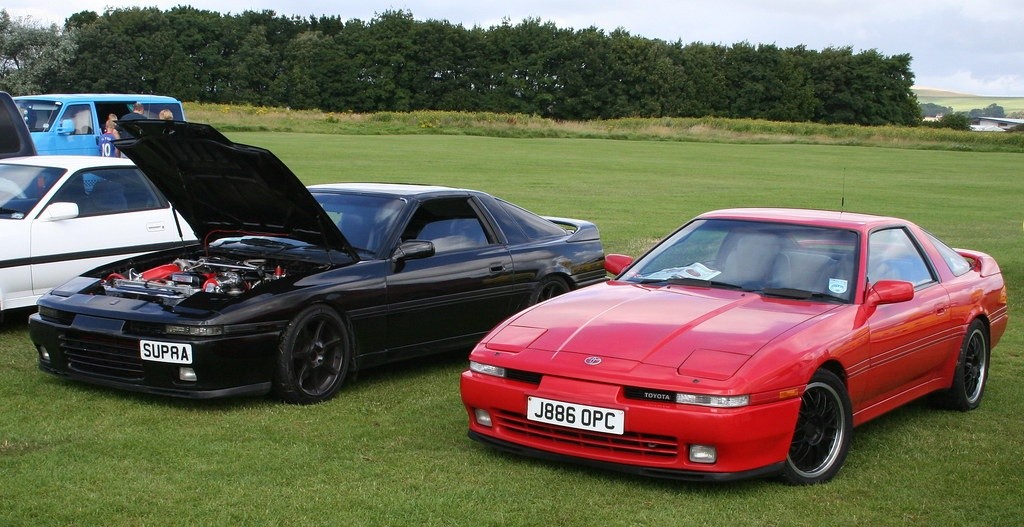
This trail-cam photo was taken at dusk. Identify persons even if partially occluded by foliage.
[159,109,173,120]
[113,102,147,139]
[98,119,115,158]
[108,114,121,158]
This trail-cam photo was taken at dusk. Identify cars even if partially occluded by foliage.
[27,117,607,399]
[0,155,197,312]
[461,207,1012,483]
[1,93,36,159]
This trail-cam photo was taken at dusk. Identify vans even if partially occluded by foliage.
[14,94,186,157]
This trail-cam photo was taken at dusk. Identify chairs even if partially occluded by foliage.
[89,181,130,214]
[722,233,778,286]
